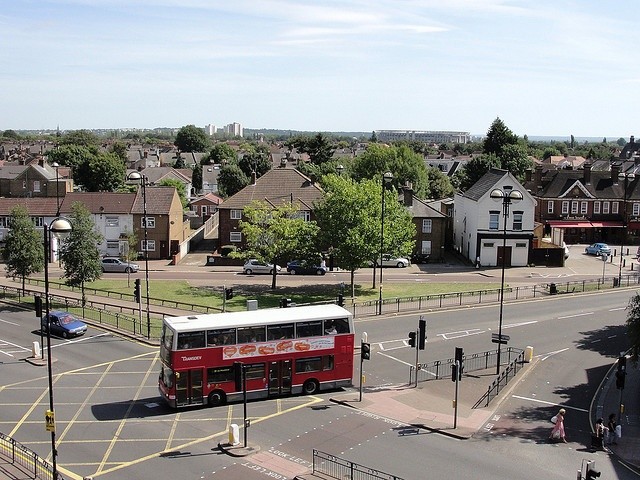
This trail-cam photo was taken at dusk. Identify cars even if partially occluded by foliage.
[368,253,408,268]
[287,260,327,275]
[100,258,140,273]
[40,310,88,339]
[243,259,282,275]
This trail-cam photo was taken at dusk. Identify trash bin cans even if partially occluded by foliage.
[550,283,556,295]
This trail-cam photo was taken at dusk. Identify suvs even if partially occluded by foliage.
[585,243,611,256]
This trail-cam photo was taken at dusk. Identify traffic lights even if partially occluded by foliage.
[361,343,370,360]
[455,347,466,381]
[409,332,416,347]
[33,295,42,317]
[134,279,140,303]
[586,460,601,480]
[338,294,345,306]
[615,357,626,390]
[226,289,233,300]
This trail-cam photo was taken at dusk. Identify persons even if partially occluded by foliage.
[223,335,229,345]
[180,340,188,349]
[250,331,256,342]
[325,323,337,335]
[548,408,568,444]
[608,413,618,447]
[207,337,218,346]
[595,418,609,451]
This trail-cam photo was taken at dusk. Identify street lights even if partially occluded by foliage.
[43,217,73,480]
[489,185,524,374]
[125,171,150,340]
[50,161,60,206]
[379,171,394,315]
[618,171,636,287]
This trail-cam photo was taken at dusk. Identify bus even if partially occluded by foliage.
[158,304,355,409]
